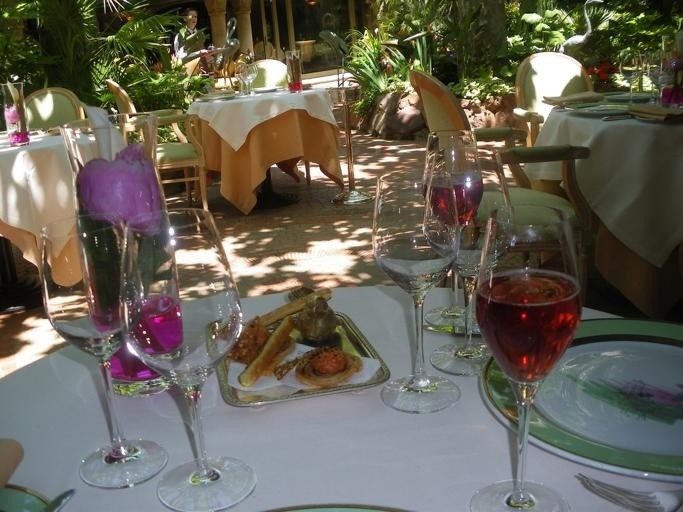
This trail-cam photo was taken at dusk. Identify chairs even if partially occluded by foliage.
[239,59,287,91]
[24,86,87,129]
[408,67,598,308]
[104,76,226,225]
[511,51,593,147]
[319,30,354,88]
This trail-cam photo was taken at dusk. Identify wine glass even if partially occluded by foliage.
[473,201,583,512]
[618,48,672,106]
[119,206,257,512]
[420,126,496,378]
[234,61,259,99]
[37,212,169,491]
[372,170,461,416]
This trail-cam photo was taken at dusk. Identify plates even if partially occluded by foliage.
[476,316,682,485]
[563,105,629,116]
[607,94,649,103]
[200,96,233,101]
[0,484,53,512]
[635,115,658,122]
[258,504,410,512]
[255,87,277,93]
[302,84,312,89]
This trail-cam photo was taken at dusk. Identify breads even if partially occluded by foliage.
[231,283,363,389]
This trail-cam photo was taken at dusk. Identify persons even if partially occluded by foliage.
[174,8,204,58]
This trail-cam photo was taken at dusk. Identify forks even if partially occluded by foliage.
[569,470,682,512]
